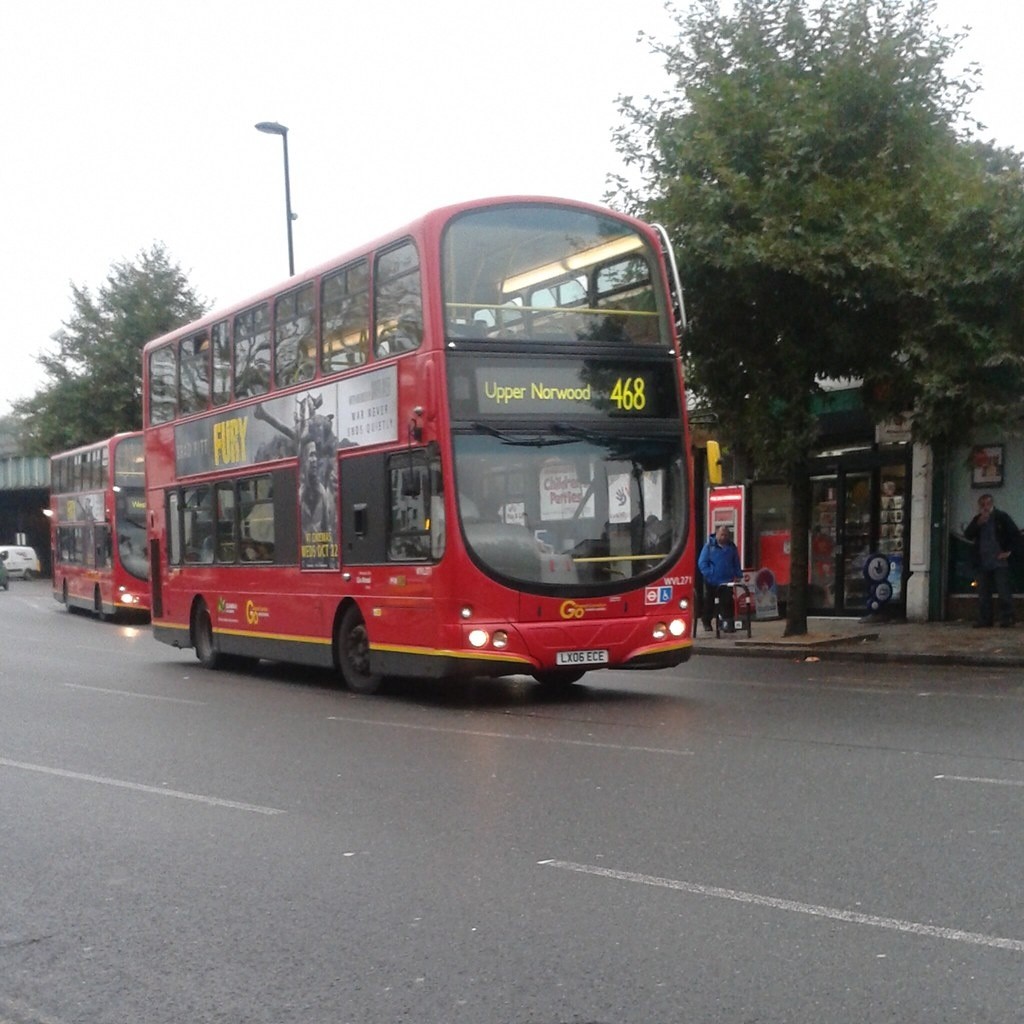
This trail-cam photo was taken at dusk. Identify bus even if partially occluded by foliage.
[143,193,725,696]
[49,430,145,620]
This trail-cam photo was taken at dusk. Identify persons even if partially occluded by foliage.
[82,499,96,521]
[699,525,744,634]
[299,439,338,570]
[429,472,494,558]
[964,494,1024,628]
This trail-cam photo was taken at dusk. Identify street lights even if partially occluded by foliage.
[256,120,295,280]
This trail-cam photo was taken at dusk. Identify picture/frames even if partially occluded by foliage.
[970,443,1005,488]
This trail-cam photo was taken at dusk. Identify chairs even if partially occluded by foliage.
[162,320,417,419]
[183,535,273,562]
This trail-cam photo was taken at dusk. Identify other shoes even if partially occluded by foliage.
[973,622,993,628]
[705,625,712,631]
[1000,614,1021,628]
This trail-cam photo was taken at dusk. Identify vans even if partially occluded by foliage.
[0,545,41,582]
[0,551,10,589]
